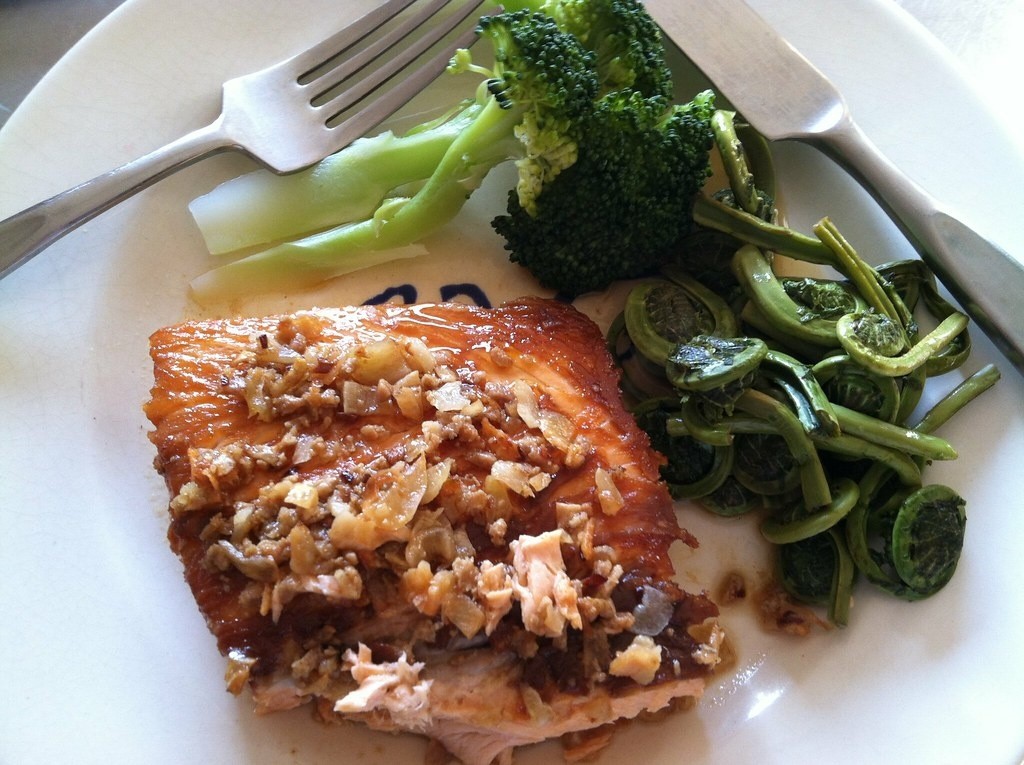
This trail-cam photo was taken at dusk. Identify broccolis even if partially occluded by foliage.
[189,0,713,305]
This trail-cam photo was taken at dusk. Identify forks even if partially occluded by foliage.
[0,0,507,283]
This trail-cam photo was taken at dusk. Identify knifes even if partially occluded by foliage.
[636,0,1024,368]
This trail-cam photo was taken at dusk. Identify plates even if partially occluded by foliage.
[0,0,1024,765]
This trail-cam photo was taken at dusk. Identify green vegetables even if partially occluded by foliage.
[602,106,1005,627]
